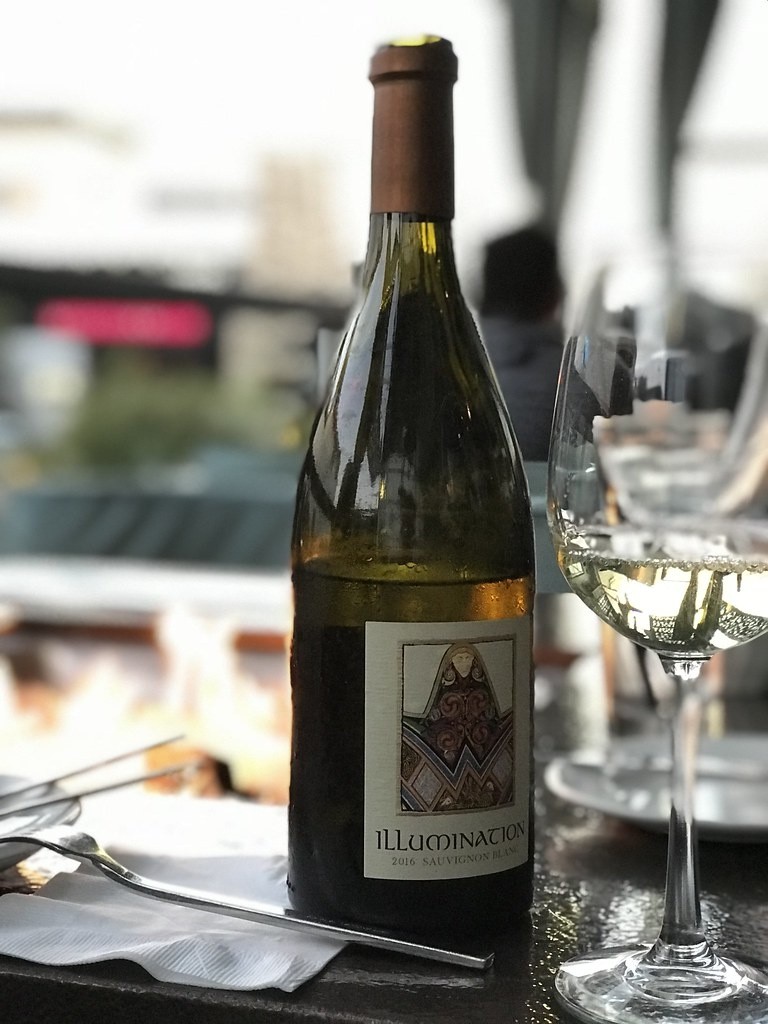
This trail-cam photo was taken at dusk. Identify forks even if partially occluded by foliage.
[0,826,495,968]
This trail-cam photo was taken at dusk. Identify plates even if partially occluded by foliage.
[544,732,768,837]
[0,775,82,869]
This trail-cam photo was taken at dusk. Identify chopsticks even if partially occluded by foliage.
[0,732,193,819]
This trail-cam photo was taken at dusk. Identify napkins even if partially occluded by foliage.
[0,842,347,993]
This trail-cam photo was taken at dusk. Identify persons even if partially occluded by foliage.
[473,231,598,458]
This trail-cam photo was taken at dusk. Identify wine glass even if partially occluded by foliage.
[547,234,768,1024]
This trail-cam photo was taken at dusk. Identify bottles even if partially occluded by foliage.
[287,33,536,967]
